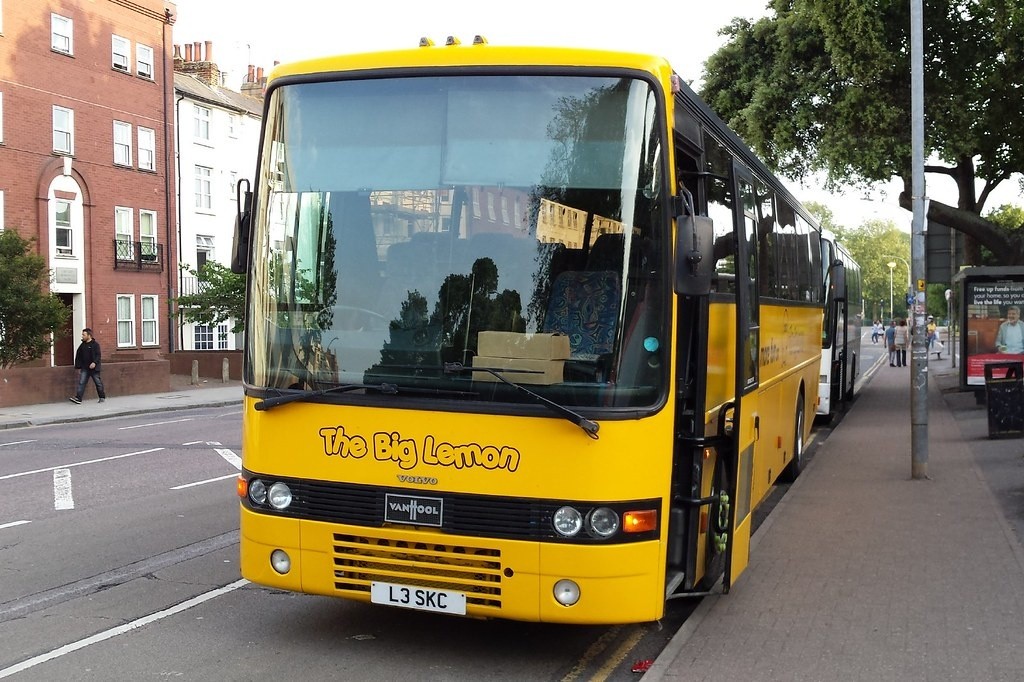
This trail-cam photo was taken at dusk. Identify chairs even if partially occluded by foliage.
[386,232,638,362]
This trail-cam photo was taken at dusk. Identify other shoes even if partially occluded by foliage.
[890,363,896,367]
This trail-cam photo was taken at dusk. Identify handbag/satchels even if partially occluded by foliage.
[890,344,896,351]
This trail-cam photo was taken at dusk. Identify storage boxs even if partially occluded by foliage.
[472,356,564,385]
[478,331,570,360]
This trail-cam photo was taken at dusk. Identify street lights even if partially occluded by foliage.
[887,262,896,323]
[882,255,912,290]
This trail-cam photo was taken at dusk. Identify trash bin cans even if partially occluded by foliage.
[982,359,1024,438]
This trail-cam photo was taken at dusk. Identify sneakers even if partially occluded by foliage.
[98,398,105,403]
[69,397,82,404]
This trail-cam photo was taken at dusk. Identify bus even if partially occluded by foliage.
[816,227,861,421]
[230,37,829,625]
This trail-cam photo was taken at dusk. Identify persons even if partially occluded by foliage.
[69,328,105,404]
[872,315,937,367]
[994,306,1024,355]
